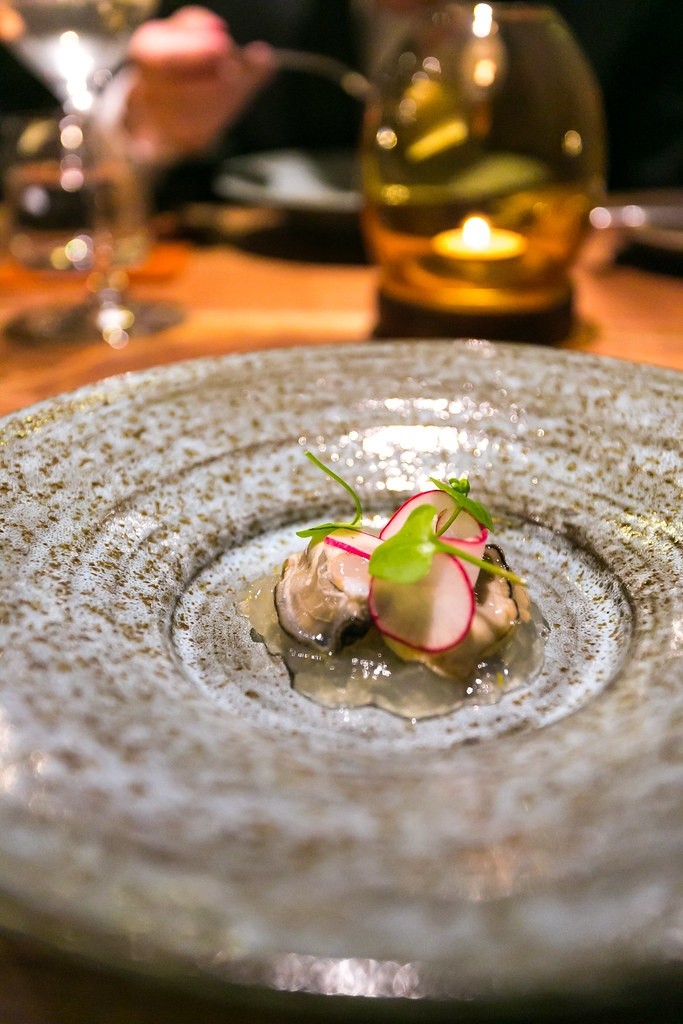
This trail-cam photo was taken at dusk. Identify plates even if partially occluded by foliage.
[589,202,683,252]
[0,336,683,1012]
[211,148,355,223]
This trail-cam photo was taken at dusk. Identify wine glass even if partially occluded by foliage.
[0,0,185,347]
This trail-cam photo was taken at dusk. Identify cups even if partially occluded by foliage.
[0,109,151,271]
[359,0,598,337]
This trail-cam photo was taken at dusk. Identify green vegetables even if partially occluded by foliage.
[295,451,528,587]
